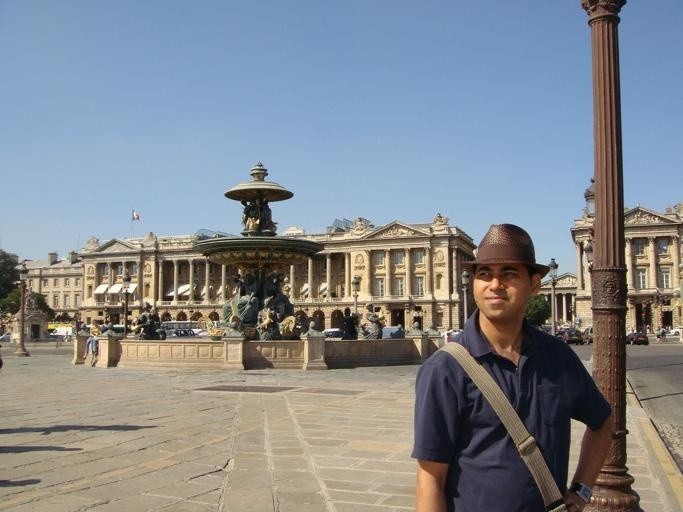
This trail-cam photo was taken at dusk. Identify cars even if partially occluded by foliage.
[669,327,683,335]
[626,332,648,345]
[557,326,593,345]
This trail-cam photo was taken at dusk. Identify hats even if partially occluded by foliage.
[460,224,550,280]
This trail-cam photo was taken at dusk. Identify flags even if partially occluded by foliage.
[132,210,140,221]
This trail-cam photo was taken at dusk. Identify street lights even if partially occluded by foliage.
[584,235,593,264]
[122,270,131,339]
[14,263,30,357]
[352,275,361,314]
[547,258,558,335]
[103,306,109,325]
[461,269,470,322]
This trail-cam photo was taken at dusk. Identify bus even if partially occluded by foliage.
[162,321,220,337]
[47,320,76,332]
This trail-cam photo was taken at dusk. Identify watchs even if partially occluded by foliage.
[569,481,593,504]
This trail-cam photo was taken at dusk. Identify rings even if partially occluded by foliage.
[575,504,580,509]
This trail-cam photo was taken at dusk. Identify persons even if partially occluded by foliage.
[389,324,405,339]
[135,196,386,341]
[411,221,615,512]
[441,327,459,343]
[661,327,666,342]
[656,329,661,344]
[64,319,115,359]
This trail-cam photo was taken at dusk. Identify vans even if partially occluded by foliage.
[49,327,77,336]
